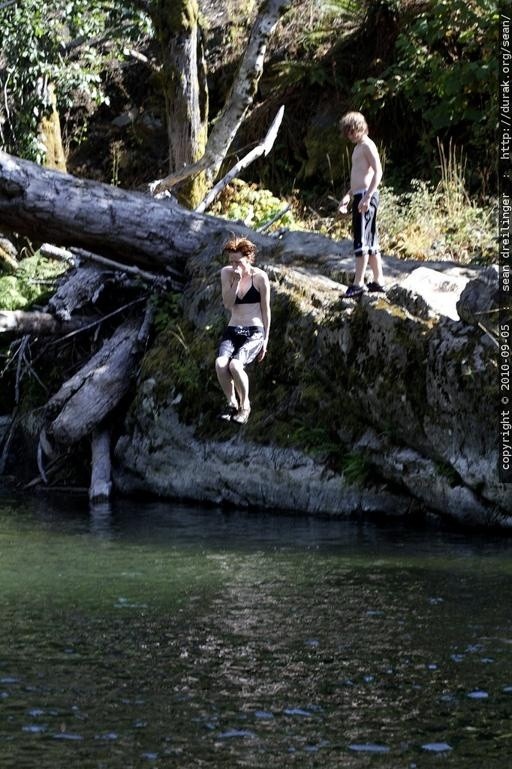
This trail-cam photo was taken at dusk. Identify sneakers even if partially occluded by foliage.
[340,282,387,299]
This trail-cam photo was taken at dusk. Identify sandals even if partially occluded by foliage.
[215,406,251,424]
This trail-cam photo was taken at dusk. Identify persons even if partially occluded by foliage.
[214,238,271,424]
[337,112,386,297]
